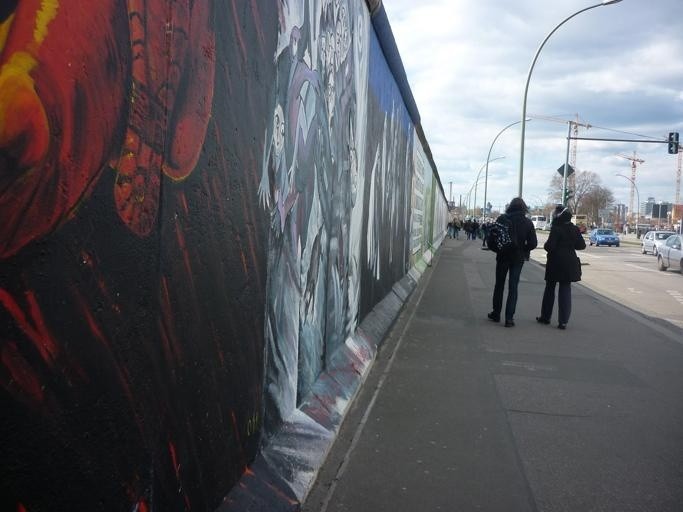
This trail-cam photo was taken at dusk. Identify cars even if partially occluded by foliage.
[588,227,619,247]
[655,235,682,274]
[640,230,675,257]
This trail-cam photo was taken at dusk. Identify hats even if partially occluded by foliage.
[504,196,526,214]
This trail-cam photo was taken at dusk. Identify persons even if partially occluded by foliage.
[446,216,497,247]
[487,197,538,327]
[536,204,586,330]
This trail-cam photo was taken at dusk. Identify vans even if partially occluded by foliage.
[637,223,655,234]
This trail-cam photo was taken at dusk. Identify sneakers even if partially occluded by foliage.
[556,323,566,330]
[534,315,550,325]
[503,318,514,328]
[488,311,502,322]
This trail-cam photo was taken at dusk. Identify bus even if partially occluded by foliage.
[569,214,587,233]
[530,215,547,230]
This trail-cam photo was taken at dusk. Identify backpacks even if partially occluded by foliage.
[481,218,513,254]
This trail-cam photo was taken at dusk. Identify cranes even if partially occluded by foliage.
[527,110,590,212]
[617,149,645,217]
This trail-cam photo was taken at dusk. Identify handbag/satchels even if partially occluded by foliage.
[571,254,583,283]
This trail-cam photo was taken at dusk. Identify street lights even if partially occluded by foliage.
[462,156,504,219]
[615,173,639,235]
[666,210,671,225]
[481,118,531,249]
[656,200,662,226]
[517,0,622,198]
